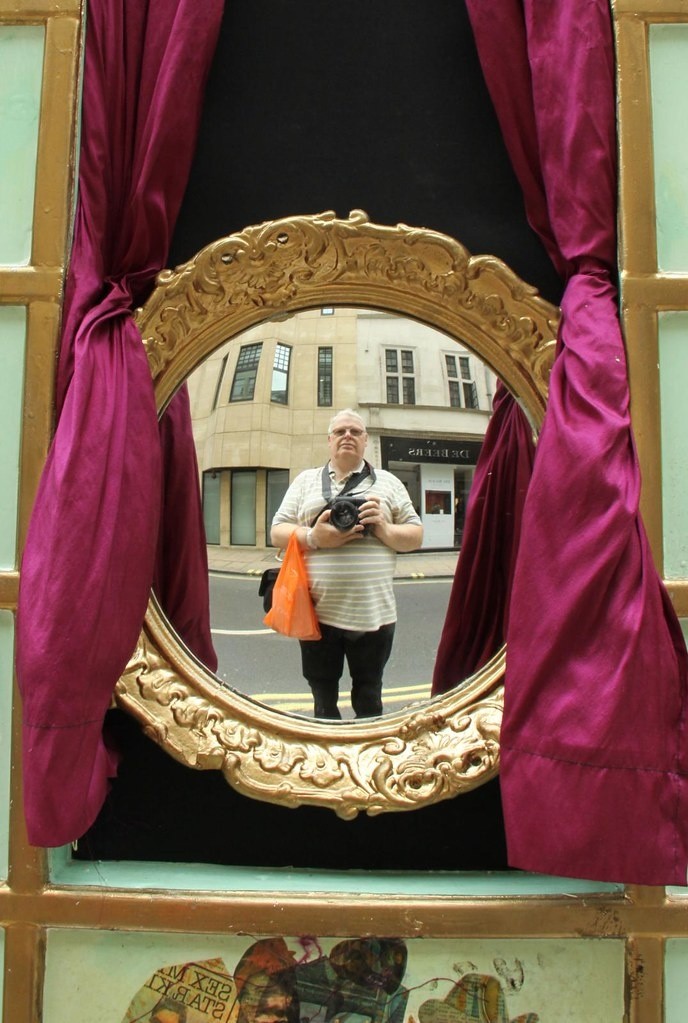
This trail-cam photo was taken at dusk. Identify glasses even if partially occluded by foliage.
[329,427,365,437]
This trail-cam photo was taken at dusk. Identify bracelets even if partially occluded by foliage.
[307,528,318,550]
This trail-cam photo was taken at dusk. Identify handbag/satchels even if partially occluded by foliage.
[258,568,280,611]
[263,533,322,640]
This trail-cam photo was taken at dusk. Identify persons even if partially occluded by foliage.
[148,998,187,1023]
[269,408,425,720]
[235,939,298,1023]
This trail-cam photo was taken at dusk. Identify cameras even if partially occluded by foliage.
[330,496,373,538]
